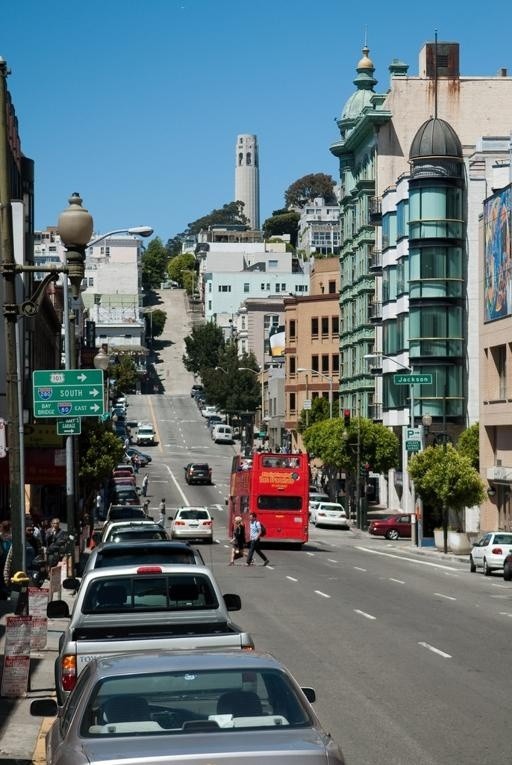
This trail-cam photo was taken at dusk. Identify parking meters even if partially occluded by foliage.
[8,570,31,614]
[25,510,91,589]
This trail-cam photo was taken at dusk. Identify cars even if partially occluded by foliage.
[369,512,411,541]
[109,382,156,463]
[62,462,217,608]
[503,556,512,582]
[307,484,350,531]
[191,383,234,444]
[27,647,347,763]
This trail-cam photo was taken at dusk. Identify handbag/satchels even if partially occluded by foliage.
[234,552,243,559]
[257,524,266,536]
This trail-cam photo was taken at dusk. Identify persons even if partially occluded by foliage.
[245,512,269,566]
[229,516,245,566]
[0,518,69,614]
[239,443,302,484]
[83,453,166,529]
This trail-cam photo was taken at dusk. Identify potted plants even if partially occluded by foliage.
[432,525,458,552]
[449,530,473,554]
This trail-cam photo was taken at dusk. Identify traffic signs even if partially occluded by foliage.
[394,373,432,384]
[32,370,106,417]
[56,418,83,435]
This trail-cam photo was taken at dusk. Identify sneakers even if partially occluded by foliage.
[264,560,269,566]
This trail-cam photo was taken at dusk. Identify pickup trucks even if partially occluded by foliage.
[43,563,254,707]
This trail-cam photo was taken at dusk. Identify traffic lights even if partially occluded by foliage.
[343,409,351,428]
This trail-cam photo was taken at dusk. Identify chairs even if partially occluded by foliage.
[96,587,128,611]
[217,693,265,715]
[170,584,200,608]
[98,695,153,722]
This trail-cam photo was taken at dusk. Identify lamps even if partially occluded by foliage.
[487,486,496,497]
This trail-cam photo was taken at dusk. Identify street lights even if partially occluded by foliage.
[343,396,365,533]
[0,134,95,626]
[423,396,448,453]
[89,343,110,421]
[214,366,228,376]
[178,269,194,299]
[296,367,334,421]
[59,224,154,568]
[238,367,268,451]
[362,354,417,546]
[166,256,174,260]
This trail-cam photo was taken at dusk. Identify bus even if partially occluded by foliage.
[223,451,310,549]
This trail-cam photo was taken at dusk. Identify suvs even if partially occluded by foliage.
[468,530,512,576]
